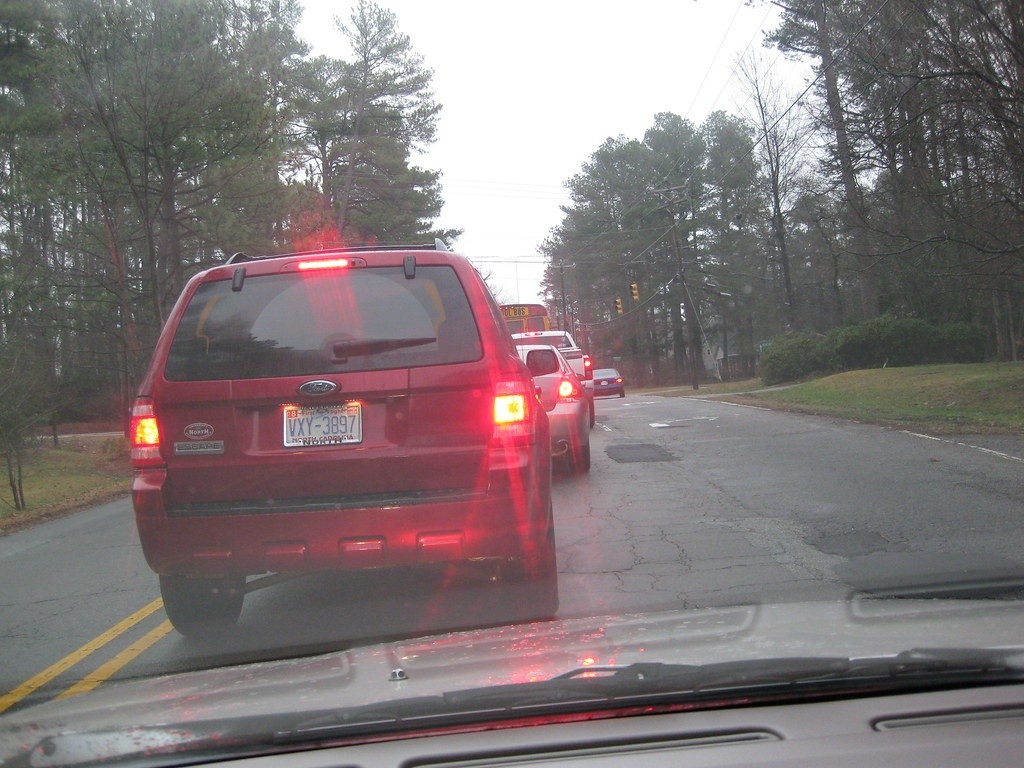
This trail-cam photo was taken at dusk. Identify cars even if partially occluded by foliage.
[591,368,624,397]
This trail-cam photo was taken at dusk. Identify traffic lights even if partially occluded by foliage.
[630,282,639,303]
[615,299,622,317]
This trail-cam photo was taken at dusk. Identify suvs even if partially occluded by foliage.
[512,343,592,475]
[125,242,565,642]
[511,328,595,429]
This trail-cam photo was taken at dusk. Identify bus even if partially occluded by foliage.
[499,305,550,333]
